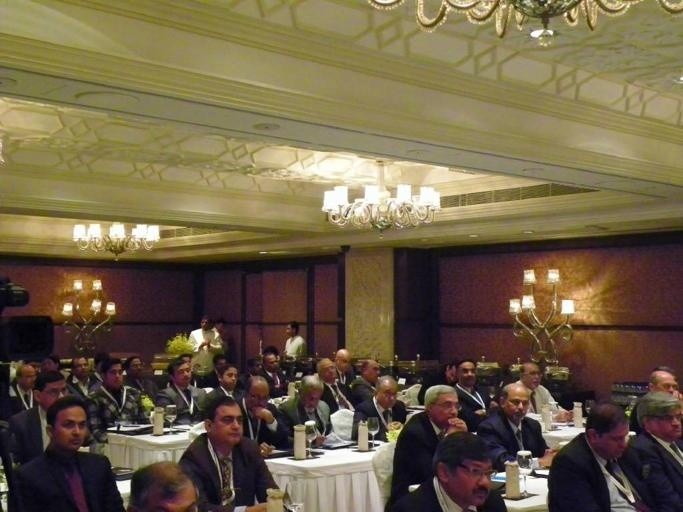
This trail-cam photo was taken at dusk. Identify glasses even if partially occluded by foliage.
[643,413,682,422]
[434,402,462,411]
[36,387,68,395]
[459,464,497,479]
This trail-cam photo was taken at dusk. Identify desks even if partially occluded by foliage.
[491,468,550,511]
[262,442,386,512]
[92,422,200,472]
[533,418,637,451]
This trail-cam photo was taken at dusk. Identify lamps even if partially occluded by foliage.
[61,279,117,354]
[509,269,576,364]
[321,158,442,240]
[72,221,160,262]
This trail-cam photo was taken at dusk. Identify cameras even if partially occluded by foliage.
[0,276,30,307]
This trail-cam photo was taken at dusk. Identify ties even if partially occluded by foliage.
[669,443,683,463]
[341,373,344,382]
[383,409,389,425]
[221,458,233,504]
[517,428,522,442]
[331,385,349,411]
[272,373,278,385]
[530,393,537,414]
[604,461,653,512]
[65,460,89,512]
[308,410,322,433]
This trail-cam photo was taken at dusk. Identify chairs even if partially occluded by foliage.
[372,442,411,511]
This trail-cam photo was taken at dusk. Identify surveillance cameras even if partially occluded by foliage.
[340,245,350,252]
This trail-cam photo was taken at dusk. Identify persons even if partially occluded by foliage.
[333,348,355,404]
[352,359,382,407]
[471,382,558,469]
[618,393,683,511]
[179,354,207,387]
[9,372,67,462]
[350,377,406,447]
[260,353,287,399]
[126,463,199,511]
[512,363,574,425]
[25,356,42,374]
[88,359,151,440]
[419,363,456,406]
[187,317,222,387]
[548,405,659,512]
[277,377,331,448]
[454,359,499,430]
[40,355,62,377]
[201,366,243,418]
[236,356,263,395]
[631,367,681,434]
[8,398,124,512]
[388,433,508,511]
[158,359,207,422]
[282,320,306,378]
[178,401,280,511]
[315,359,355,415]
[393,387,466,498]
[65,357,100,402]
[240,374,290,457]
[207,318,228,360]
[207,354,226,386]
[8,366,36,412]
[123,355,159,401]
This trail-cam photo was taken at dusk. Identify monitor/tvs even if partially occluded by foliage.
[0,316,55,362]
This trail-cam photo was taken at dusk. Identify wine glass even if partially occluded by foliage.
[166,404,177,435]
[368,417,379,450]
[517,451,532,497]
[305,424,317,459]
[550,404,559,430]
